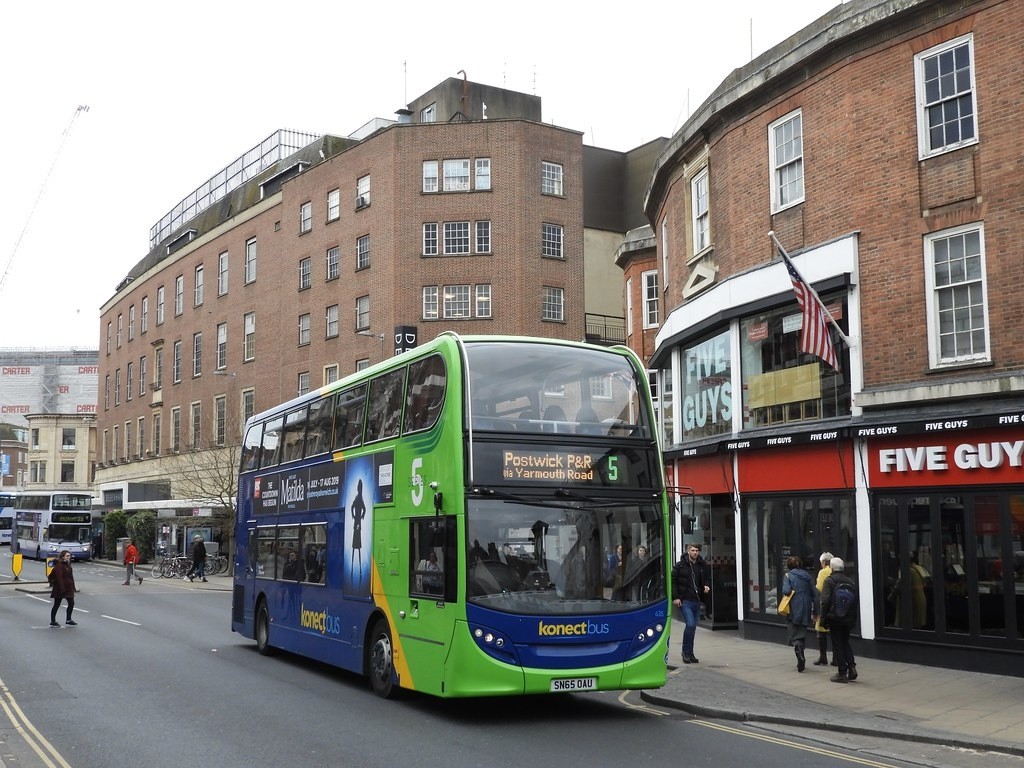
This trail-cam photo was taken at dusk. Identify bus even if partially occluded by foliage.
[230,330,697,699]
[10,491,95,563]
[0,491,16,544]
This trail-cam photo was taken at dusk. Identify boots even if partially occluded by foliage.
[831,654,838,666]
[794,644,806,673]
[815,639,828,665]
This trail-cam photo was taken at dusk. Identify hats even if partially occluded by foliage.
[194,535,200,538]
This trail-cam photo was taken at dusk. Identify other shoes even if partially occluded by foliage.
[74,589,80,592]
[188,574,194,582]
[138,577,143,585]
[201,577,208,582]
[122,581,130,585]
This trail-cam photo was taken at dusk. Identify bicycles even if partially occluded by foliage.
[151,551,228,579]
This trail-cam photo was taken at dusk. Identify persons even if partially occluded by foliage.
[887,551,927,628]
[183,535,208,583]
[782,552,858,683]
[499,545,528,564]
[422,552,440,594]
[48,550,77,628]
[91,532,103,560]
[603,544,647,606]
[671,543,711,663]
[122,540,143,586]
[255,547,327,582]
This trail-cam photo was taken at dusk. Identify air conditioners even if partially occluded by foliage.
[355,197,364,208]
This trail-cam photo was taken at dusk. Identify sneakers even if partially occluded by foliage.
[66,621,77,625]
[831,673,848,683]
[848,668,858,680]
[50,622,61,627]
[682,652,698,663]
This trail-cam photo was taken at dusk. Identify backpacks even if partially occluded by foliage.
[829,576,854,621]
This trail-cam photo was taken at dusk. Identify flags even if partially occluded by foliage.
[778,248,838,372]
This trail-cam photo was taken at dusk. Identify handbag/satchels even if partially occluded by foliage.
[48,562,66,587]
[778,573,795,616]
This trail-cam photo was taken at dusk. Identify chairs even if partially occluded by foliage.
[246,405,601,471]
